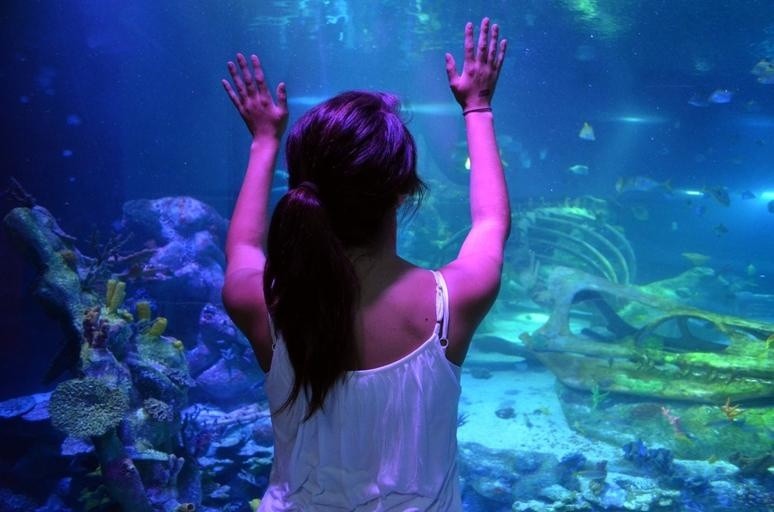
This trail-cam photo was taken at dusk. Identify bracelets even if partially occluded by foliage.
[461,107,492,117]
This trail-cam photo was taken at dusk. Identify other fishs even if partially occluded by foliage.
[0,0,773,511]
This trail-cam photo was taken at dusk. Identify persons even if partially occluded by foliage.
[219,16,508,512]
